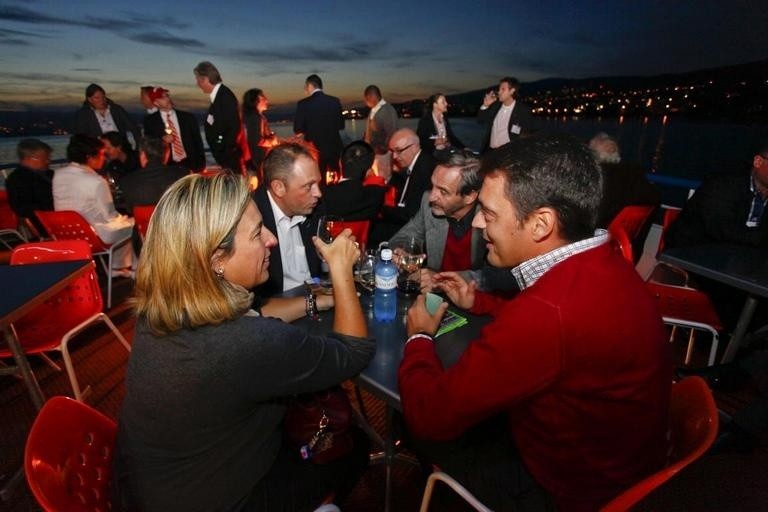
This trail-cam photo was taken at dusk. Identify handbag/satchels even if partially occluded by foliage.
[285,388,353,467]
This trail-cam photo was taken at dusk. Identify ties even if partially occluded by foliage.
[167,115,184,158]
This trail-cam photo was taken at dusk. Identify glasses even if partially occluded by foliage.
[162,94,169,98]
[390,144,412,154]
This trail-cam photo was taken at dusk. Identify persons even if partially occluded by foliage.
[7,61,250,293]
[315,130,510,292]
[475,77,534,168]
[396,135,676,511]
[696,139,766,268]
[416,93,467,157]
[294,74,345,168]
[585,129,659,222]
[364,84,399,148]
[250,143,332,297]
[241,88,278,166]
[112,168,376,510]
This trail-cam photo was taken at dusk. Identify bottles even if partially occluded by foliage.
[374,249,398,323]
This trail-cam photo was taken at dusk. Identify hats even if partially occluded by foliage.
[149,86,169,99]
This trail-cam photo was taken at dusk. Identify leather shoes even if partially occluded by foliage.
[112,268,133,277]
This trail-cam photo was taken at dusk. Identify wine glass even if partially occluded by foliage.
[358,255,375,307]
[164,120,174,136]
[315,214,343,295]
[398,236,423,292]
